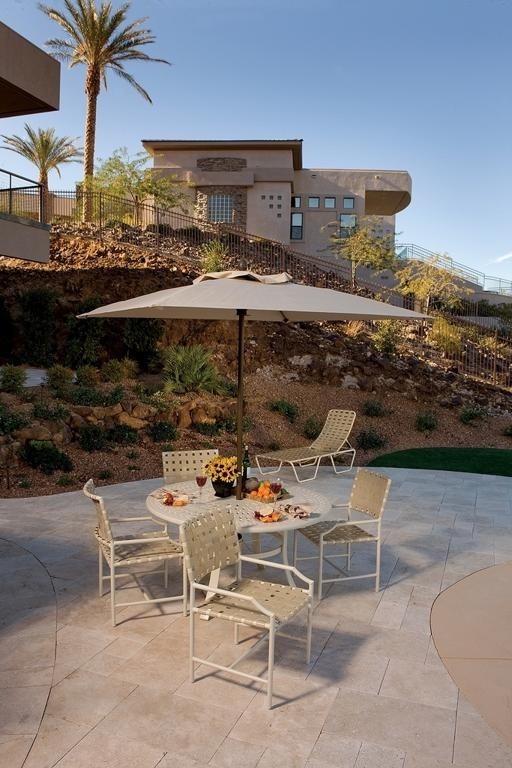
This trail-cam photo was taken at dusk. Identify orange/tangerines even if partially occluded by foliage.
[250,480,270,498]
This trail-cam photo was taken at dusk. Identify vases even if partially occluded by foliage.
[211,477,235,498]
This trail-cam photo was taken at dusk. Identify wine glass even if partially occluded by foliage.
[195,470,208,499]
[269,477,282,510]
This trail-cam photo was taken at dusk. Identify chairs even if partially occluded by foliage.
[161,449,220,484]
[179,503,314,709]
[81,479,188,627]
[254,408,358,483]
[292,466,391,599]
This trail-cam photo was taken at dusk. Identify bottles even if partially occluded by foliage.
[243,445,251,481]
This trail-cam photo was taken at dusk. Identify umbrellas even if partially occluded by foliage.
[74,273,437,500]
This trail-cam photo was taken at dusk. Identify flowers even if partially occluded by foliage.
[201,455,242,483]
